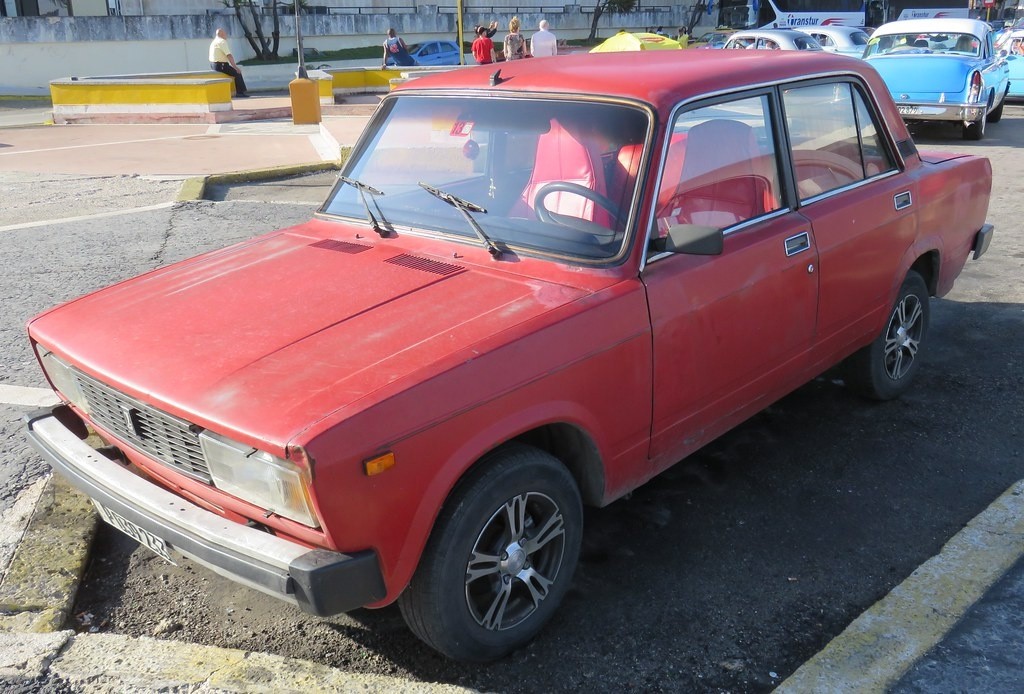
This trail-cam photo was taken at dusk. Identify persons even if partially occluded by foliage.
[474,20,499,41]
[471,26,497,65]
[503,16,528,63]
[380,29,422,70]
[208,27,251,98]
[530,20,558,57]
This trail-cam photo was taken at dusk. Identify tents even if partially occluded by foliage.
[585,30,683,53]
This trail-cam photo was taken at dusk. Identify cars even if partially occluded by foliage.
[697,30,750,49]
[853,17,1024,103]
[793,25,871,60]
[860,18,1011,140]
[720,29,824,52]
[382,40,468,66]
[687,24,744,49]
[22,49,993,661]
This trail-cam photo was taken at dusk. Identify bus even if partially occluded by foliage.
[866,0,970,30]
[708,0,865,30]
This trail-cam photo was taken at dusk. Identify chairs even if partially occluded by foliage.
[650,119,775,239]
[507,112,611,230]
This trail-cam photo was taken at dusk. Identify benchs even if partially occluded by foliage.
[612,131,882,235]
[48,69,237,113]
[296,65,484,105]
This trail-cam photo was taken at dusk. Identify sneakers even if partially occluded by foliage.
[236,92,250,97]
